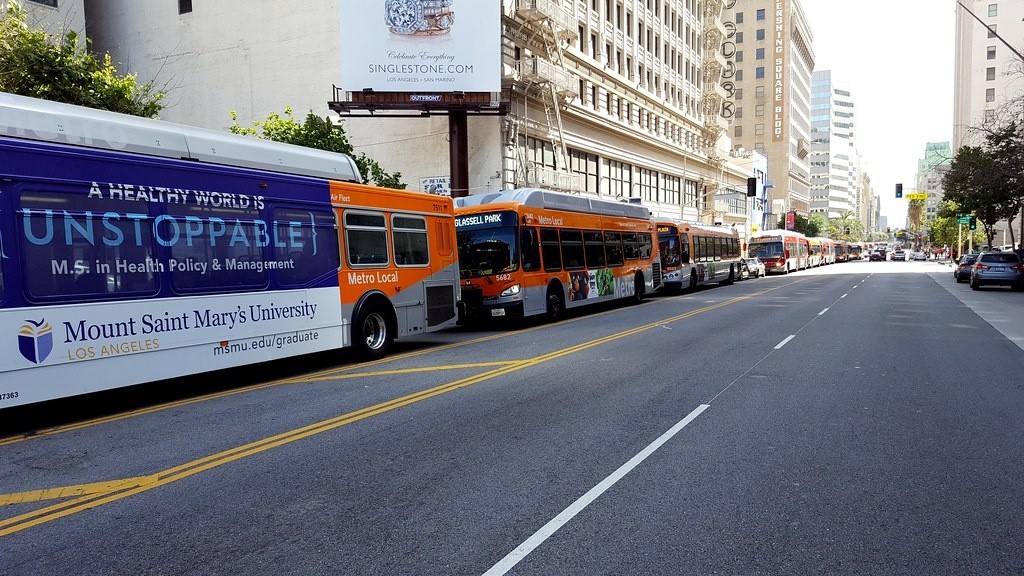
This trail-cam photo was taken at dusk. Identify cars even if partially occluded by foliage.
[748,258,766,278]
[969,251,1024,289]
[741,258,750,279]
[954,254,979,284]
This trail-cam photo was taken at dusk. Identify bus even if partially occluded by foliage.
[0,92,460,410]
[805,237,926,264]
[743,230,810,275]
[656,220,742,292]
[451,188,666,323]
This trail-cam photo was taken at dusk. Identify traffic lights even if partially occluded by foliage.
[918,236,921,243]
[895,184,903,198]
[887,227,890,233]
[970,216,977,230]
